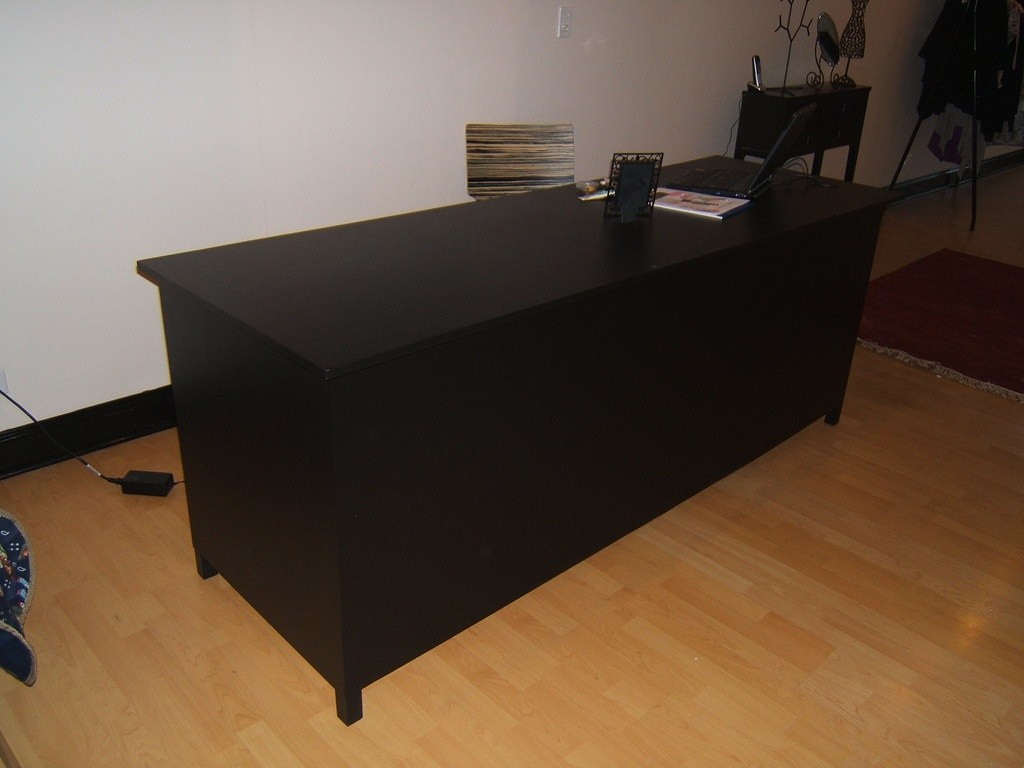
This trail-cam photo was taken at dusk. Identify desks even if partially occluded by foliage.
[734,81,872,180]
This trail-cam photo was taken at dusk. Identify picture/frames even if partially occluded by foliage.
[603,152,665,218]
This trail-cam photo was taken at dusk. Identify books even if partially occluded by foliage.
[647,182,758,219]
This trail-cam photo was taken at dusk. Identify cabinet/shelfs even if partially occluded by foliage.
[135,154,894,727]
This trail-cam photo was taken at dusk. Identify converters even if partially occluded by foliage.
[121,469,175,497]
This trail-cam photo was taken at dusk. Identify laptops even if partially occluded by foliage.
[665,100,819,200]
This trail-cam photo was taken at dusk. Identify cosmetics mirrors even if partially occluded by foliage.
[806,11,846,92]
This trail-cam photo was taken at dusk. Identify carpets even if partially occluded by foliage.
[857,247,1024,404]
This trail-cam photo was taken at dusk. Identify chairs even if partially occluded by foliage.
[465,123,576,201]
